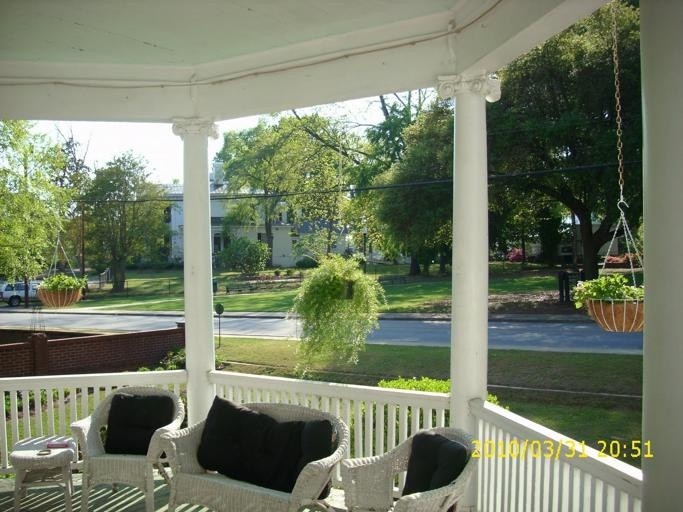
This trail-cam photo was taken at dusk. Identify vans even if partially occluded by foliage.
[0,281,45,306]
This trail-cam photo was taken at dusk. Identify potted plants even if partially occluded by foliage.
[35,273,87,307]
[569,272,646,333]
[284,247,388,381]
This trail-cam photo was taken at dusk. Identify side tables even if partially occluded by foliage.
[11,435,76,511]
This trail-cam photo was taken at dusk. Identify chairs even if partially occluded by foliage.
[69,386,185,512]
[341,428,477,512]
[160,402,349,512]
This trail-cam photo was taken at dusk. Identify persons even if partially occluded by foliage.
[552,232,578,305]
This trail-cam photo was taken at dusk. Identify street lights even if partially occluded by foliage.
[361,226,367,274]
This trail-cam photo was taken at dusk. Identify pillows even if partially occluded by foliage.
[198,395,332,502]
[104,393,173,456]
[400,431,470,495]
